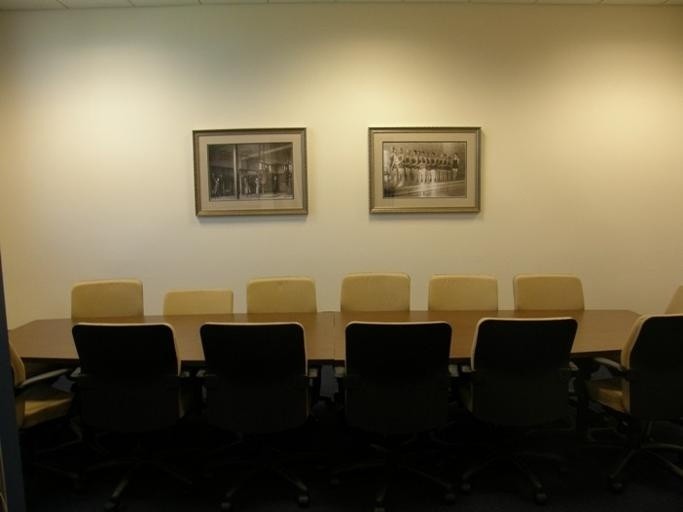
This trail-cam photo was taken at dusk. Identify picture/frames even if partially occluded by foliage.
[192,128,309,218]
[366,126,482,217]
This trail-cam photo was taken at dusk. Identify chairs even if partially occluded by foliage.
[163,289,233,315]
[73,323,190,512]
[459,317,576,506]
[573,313,683,494]
[340,273,411,311]
[332,319,459,512]
[6,337,82,494]
[198,320,314,511]
[72,283,143,318]
[515,275,584,314]
[246,280,315,316]
[663,287,683,316]
[428,277,498,312]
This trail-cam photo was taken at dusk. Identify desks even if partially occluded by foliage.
[6,310,651,368]
